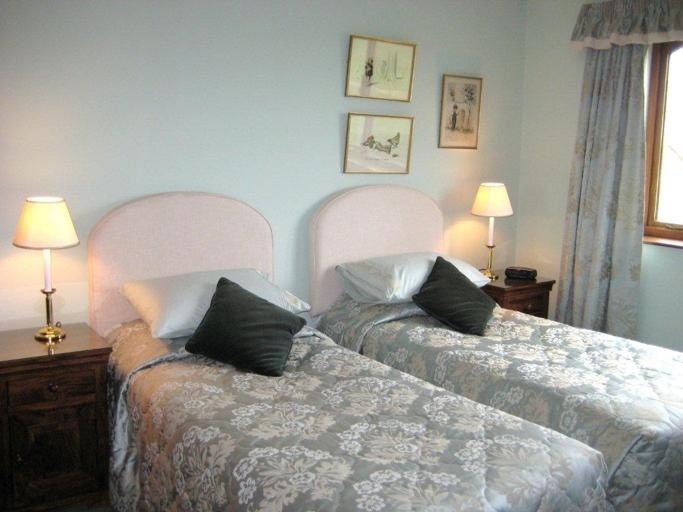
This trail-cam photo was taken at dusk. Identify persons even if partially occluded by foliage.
[450,105,457,130]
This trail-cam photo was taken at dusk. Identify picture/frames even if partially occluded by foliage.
[342,34,417,102]
[340,112,414,174]
[438,71,481,150]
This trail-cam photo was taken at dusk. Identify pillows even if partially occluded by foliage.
[409,255,497,337]
[118,267,311,338]
[334,250,493,311]
[184,275,309,379]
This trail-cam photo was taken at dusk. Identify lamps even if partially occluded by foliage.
[469,181,513,282]
[12,195,80,345]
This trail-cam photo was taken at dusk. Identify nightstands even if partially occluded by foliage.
[0,322,115,512]
[477,266,556,320]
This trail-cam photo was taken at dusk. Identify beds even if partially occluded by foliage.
[306,181,682,512]
[79,190,608,512]
